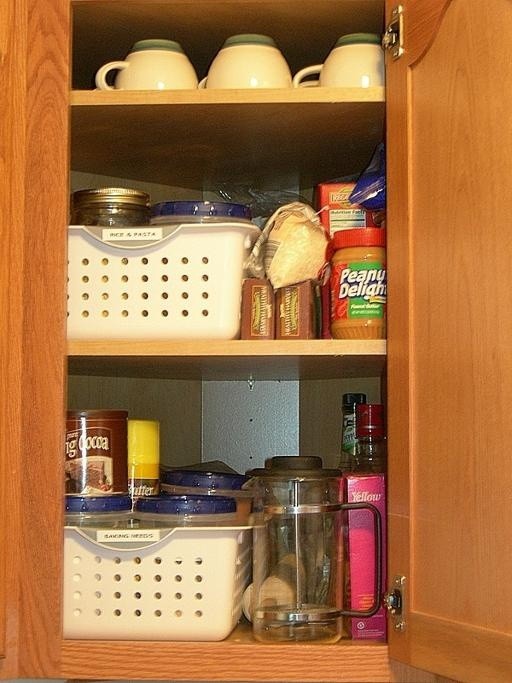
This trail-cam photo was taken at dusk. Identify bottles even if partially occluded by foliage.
[148,200,253,227]
[67,180,152,227]
[336,393,386,473]
[252,456,384,646]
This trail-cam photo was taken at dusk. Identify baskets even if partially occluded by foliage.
[60,524,255,644]
[67,222,264,341]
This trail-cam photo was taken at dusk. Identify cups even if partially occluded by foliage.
[291,32,386,88]
[95,39,199,89]
[197,35,293,89]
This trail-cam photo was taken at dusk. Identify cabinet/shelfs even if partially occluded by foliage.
[0,0,512,683]
